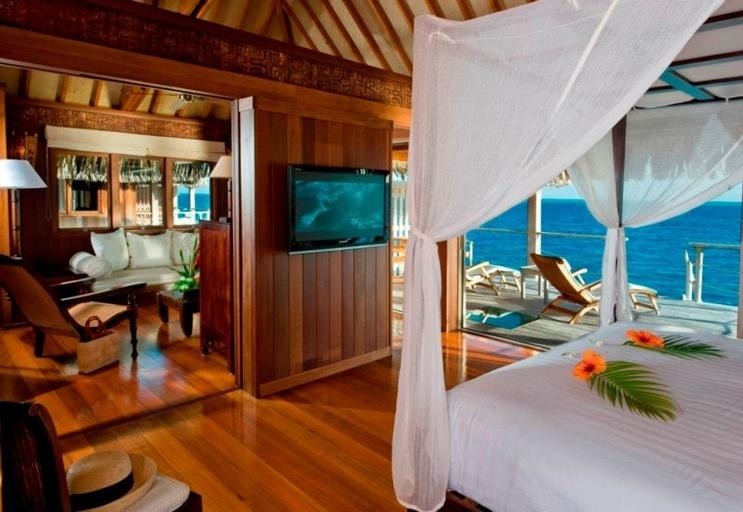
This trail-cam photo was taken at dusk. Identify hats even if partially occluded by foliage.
[66,450,158,512]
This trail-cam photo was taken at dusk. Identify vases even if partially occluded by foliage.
[183,289,200,300]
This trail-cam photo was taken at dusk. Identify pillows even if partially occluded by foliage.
[69,228,200,280]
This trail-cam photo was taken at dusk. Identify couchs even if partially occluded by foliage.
[52,228,200,294]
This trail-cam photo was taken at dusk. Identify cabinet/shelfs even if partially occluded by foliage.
[199,220,234,374]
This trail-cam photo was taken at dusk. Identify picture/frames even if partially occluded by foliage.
[32,240,57,265]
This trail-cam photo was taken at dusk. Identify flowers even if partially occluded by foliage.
[621,330,727,359]
[573,350,684,421]
[159,237,201,292]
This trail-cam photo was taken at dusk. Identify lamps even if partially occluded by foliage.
[0,159,47,260]
[209,156,232,223]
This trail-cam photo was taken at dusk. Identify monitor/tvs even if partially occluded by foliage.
[286,164,390,255]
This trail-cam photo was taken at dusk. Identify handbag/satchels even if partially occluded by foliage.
[76,316,119,376]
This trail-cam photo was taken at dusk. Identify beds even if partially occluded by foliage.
[390,0,742,512]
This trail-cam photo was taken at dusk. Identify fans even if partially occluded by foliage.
[125,92,230,112]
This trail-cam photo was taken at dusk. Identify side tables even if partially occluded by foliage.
[520,264,549,305]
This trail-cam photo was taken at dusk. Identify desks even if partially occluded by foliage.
[156,290,200,337]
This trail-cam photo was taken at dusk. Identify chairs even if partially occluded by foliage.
[0,254,138,360]
[530,253,658,325]
[1,401,202,511]
[465,261,520,295]
